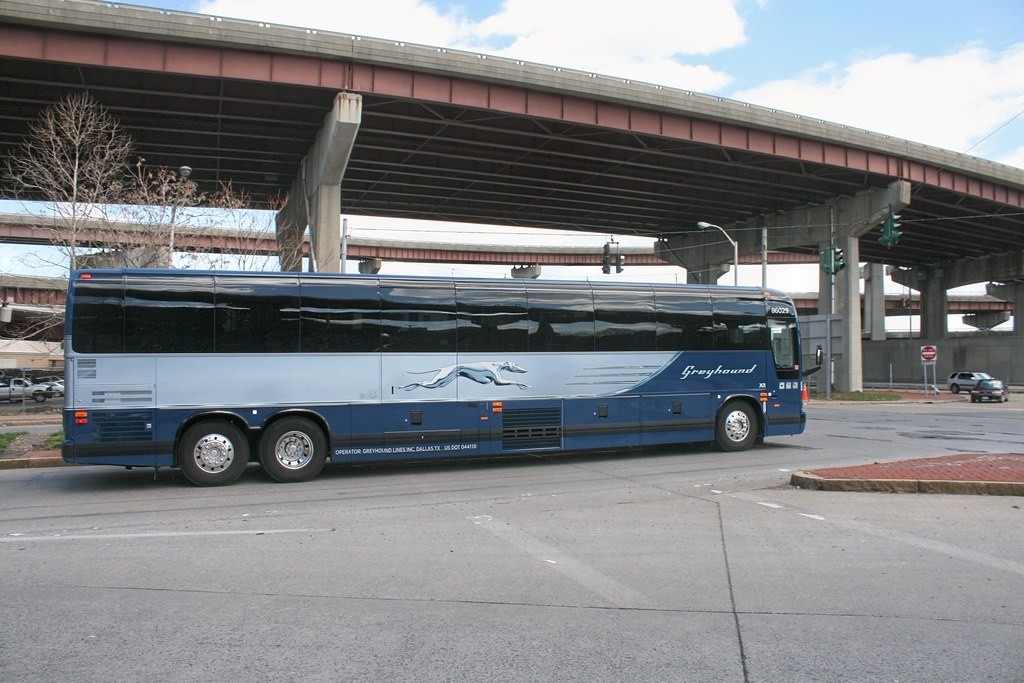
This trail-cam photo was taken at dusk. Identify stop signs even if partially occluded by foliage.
[921,346,936,361]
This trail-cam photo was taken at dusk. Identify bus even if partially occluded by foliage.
[60,269,826,485]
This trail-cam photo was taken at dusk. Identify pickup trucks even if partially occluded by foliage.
[0,377,53,402]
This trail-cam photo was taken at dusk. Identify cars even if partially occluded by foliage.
[972,378,1011,402]
[35,380,65,397]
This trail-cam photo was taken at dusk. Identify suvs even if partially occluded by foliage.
[948,370,993,394]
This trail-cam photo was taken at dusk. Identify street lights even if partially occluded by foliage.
[696,221,740,286]
[168,164,194,268]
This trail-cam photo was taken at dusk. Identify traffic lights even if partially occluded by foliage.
[878,220,890,246]
[892,213,904,242]
[833,247,845,271]
[618,255,624,273]
[818,249,830,272]
[601,245,611,274]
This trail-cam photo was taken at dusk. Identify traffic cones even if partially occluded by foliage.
[802,379,809,400]
